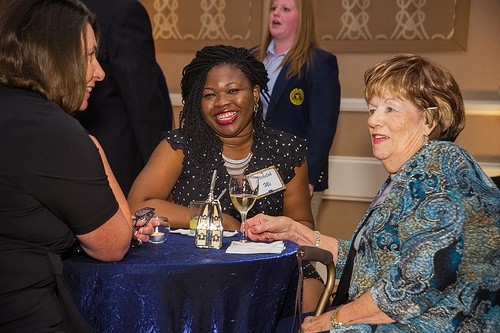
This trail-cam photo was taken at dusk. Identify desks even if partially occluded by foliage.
[65,225,302,333]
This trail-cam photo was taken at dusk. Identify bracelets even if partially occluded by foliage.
[331,305,344,326]
[314,231,320,246]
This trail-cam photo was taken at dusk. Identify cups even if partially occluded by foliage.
[189,201,207,234]
[146,217,170,244]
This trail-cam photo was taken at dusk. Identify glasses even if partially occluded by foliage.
[132,207,155,233]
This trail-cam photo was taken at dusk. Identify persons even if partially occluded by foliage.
[0,0,133,333]
[248,0,341,227]
[60,0,175,199]
[240,53,500,333]
[126,44,315,232]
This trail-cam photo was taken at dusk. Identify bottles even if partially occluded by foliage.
[195,196,223,249]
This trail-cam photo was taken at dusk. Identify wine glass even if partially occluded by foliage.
[228,175,259,245]
